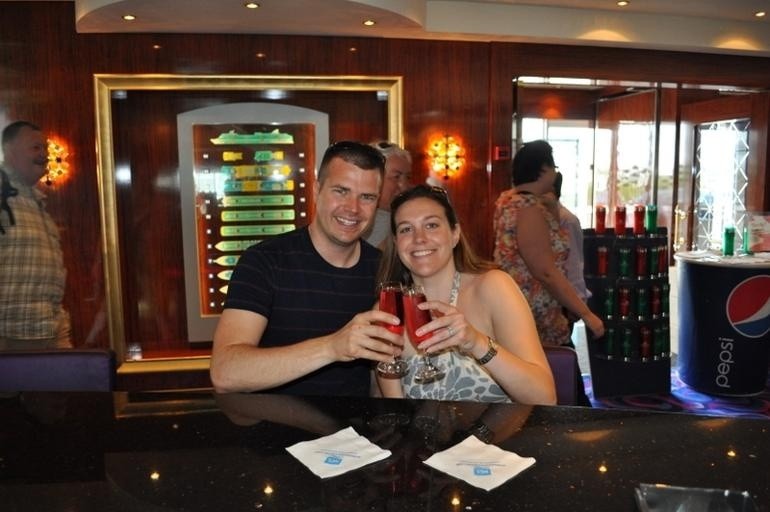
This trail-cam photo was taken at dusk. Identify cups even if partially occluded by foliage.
[742,226,755,256]
[593,202,671,363]
[723,227,735,256]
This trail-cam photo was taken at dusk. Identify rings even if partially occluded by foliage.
[446,324,456,340]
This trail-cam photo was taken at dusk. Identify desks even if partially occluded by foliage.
[674,250,769,398]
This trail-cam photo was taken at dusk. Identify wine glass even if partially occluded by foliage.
[399,286,445,384]
[374,281,410,375]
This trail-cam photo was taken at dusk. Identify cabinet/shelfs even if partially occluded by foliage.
[581,228,671,395]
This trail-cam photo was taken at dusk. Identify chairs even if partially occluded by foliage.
[2,349,113,435]
[545,346,578,406]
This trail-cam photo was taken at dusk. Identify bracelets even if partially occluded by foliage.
[473,418,496,442]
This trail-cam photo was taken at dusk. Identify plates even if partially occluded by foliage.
[633,481,758,512]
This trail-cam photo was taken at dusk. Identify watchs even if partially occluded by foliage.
[475,334,499,367]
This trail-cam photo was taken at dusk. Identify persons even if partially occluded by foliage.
[492,141,605,407]
[208,138,409,398]
[539,171,593,340]
[0,120,73,355]
[414,397,531,500]
[370,182,559,408]
[217,396,412,506]
[358,143,414,253]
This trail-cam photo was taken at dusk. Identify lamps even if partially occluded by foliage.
[424,131,465,180]
[39,139,69,186]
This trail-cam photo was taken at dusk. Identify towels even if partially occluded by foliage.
[285,427,392,478]
[423,435,537,492]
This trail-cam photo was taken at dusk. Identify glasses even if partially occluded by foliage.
[390,186,451,209]
[376,142,393,150]
[323,141,386,166]
